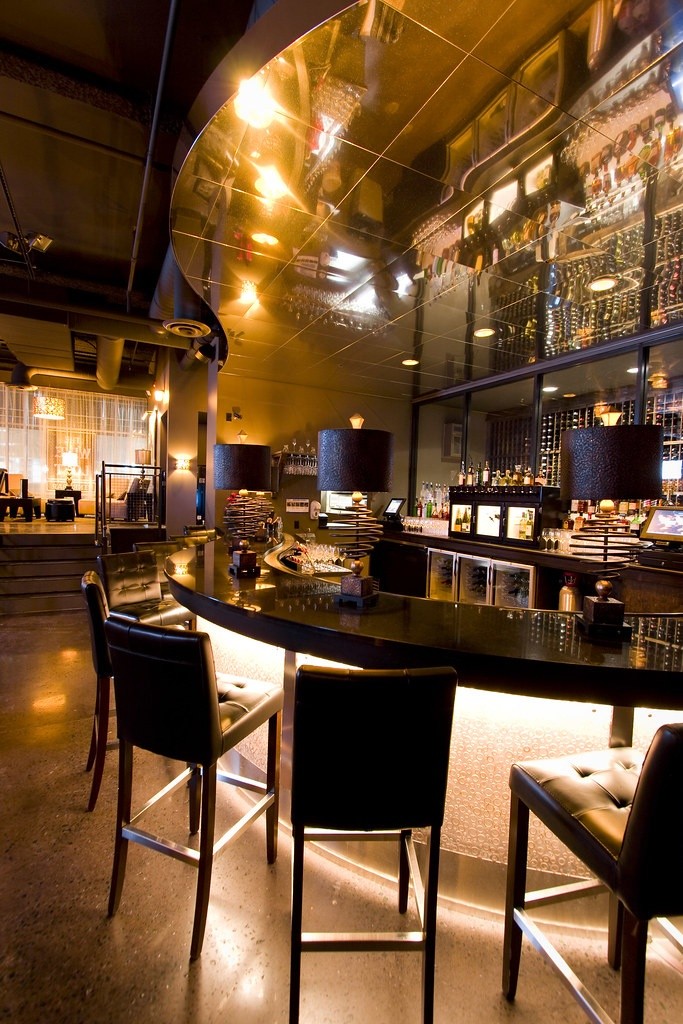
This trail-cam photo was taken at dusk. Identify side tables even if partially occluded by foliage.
[55,490,81,516]
[126,493,153,522]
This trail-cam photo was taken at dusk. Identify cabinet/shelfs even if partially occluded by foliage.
[367,536,428,599]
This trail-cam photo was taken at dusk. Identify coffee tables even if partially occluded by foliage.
[45,498,75,522]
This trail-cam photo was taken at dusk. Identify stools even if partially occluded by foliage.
[502,722,683,1024]
[133,541,182,600]
[167,535,210,552]
[103,612,284,958]
[80,570,186,812]
[289,664,457,1024]
[96,550,197,632]
[184,528,217,542]
[183,525,206,535]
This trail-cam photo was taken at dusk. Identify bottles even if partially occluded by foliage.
[491,469,512,485]
[454,507,470,533]
[458,460,490,486]
[519,511,534,540]
[417,481,449,521]
[512,467,534,486]
[488,513,500,523]
[534,468,545,485]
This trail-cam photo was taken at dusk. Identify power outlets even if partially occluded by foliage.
[294,520,300,529]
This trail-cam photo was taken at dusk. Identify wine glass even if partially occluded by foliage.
[542,528,573,555]
[282,438,319,476]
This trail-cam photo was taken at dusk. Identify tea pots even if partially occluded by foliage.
[306,540,347,570]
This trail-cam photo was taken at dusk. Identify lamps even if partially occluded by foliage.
[213,430,275,554]
[175,454,190,473]
[134,449,151,492]
[652,377,670,389]
[33,376,64,420]
[594,401,611,417]
[662,460,683,506]
[558,406,664,642]
[62,451,77,490]
[317,412,395,576]
[26,231,55,254]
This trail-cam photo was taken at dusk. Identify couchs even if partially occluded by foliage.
[0,473,46,517]
[78,477,150,521]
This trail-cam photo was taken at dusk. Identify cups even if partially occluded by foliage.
[298,445,304,453]
[310,446,317,454]
[282,444,289,452]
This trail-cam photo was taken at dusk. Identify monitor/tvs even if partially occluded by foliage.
[639,507,683,543]
[383,498,406,516]
